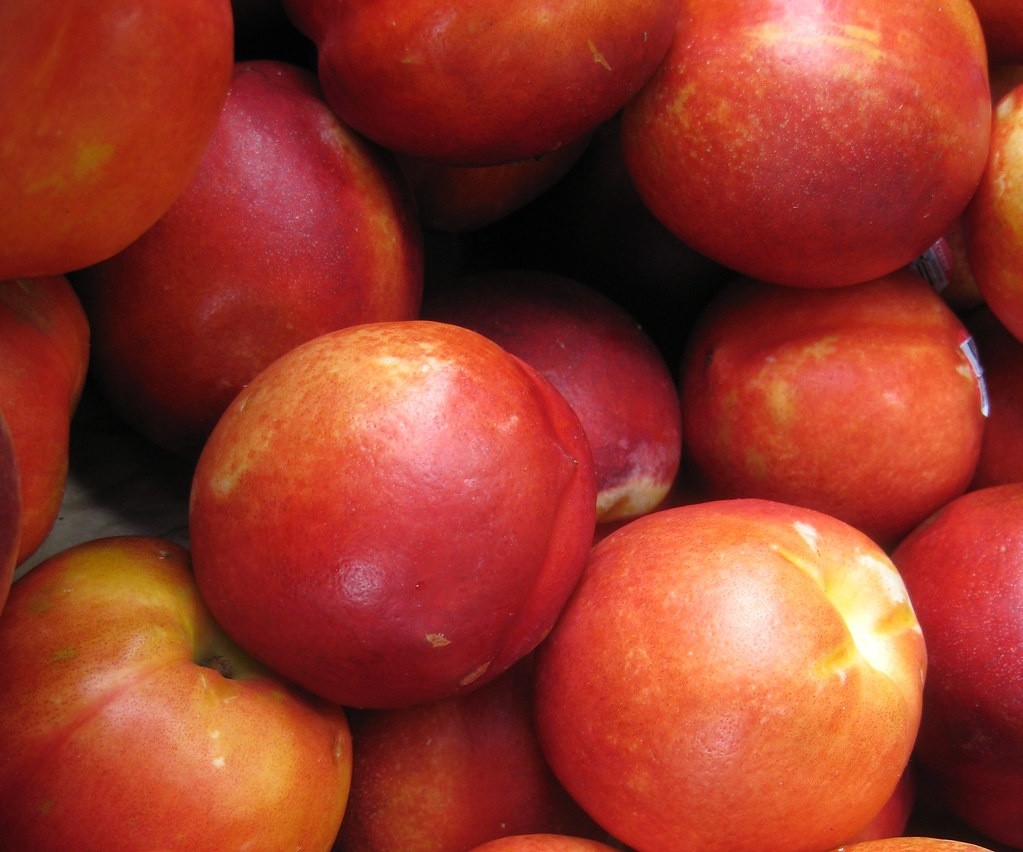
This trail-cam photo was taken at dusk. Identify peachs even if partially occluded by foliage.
[0,0,1023,852]
